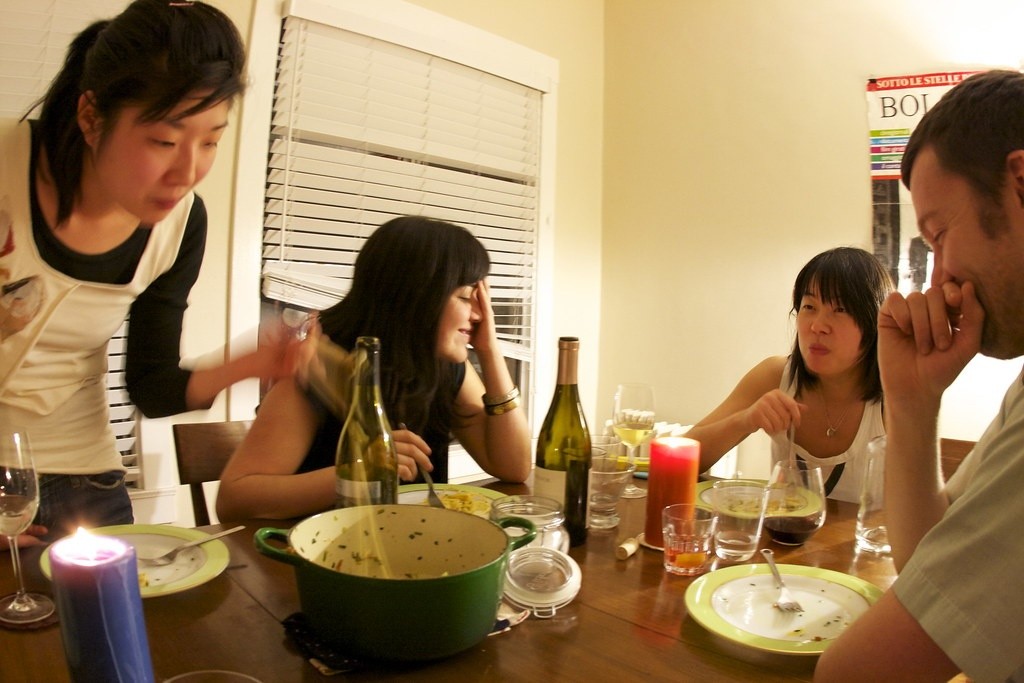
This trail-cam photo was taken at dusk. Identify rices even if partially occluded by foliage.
[414,492,489,515]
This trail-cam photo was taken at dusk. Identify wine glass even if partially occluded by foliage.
[0,425,54,625]
[612,382,656,499]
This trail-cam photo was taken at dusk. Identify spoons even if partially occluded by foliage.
[399,422,446,510]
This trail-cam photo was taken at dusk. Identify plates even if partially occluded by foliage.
[683,563,886,655]
[397,483,507,518]
[36,523,230,600]
[695,478,821,519]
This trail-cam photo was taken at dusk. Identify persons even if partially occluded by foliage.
[811,72,1024,683]
[0,0,323,548]
[679,246,897,513]
[210,214,532,521]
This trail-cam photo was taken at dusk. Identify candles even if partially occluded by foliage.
[47,530,154,683]
[644,439,701,548]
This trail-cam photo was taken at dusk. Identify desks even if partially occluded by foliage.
[0,470,901,683]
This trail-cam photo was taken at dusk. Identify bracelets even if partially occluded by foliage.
[482,385,520,406]
[485,396,521,414]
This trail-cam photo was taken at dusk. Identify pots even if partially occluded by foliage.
[252,504,537,664]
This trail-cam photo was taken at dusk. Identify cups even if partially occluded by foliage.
[711,480,769,561]
[589,433,637,530]
[854,435,891,554]
[662,505,720,575]
[764,461,826,547]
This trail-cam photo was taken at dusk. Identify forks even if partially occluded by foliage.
[138,526,245,567]
[760,549,805,614]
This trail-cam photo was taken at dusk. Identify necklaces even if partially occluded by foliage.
[820,389,848,438]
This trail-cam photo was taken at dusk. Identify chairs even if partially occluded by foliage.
[172,420,254,526]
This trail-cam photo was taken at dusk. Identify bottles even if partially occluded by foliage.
[335,337,400,508]
[489,494,570,563]
[533,337,592,548]
